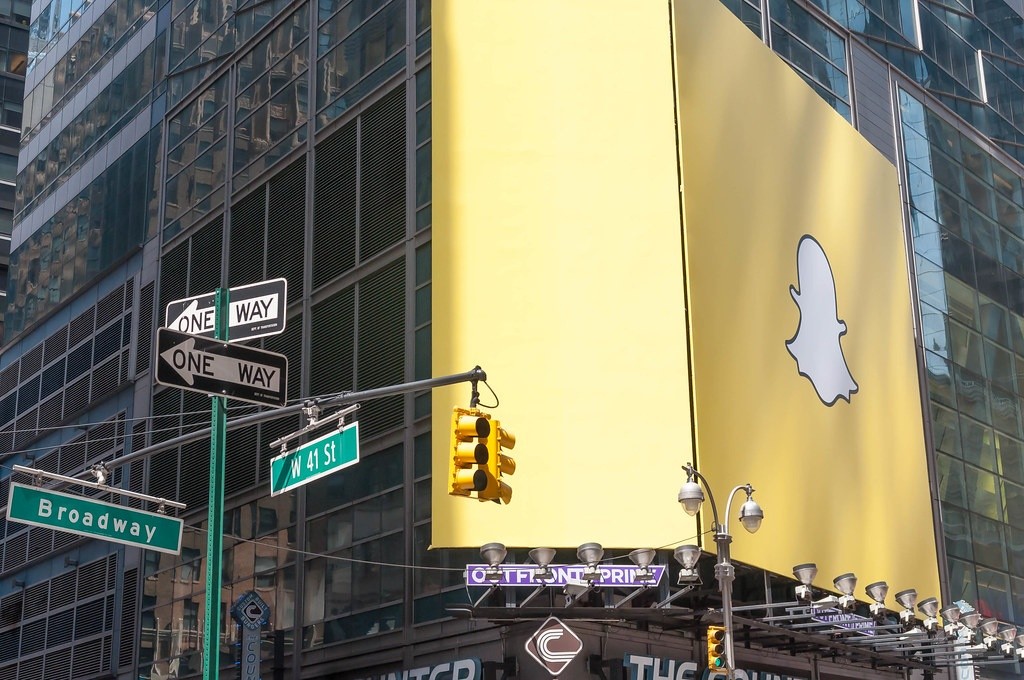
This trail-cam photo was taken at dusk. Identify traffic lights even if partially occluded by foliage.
[447,405,493,503]
[480,418,517,506]
[705,625,726,672]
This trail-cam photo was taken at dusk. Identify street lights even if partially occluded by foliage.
[676,462,765,680]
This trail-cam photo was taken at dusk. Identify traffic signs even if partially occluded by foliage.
[155,325,288,407]
[165,278,289,344]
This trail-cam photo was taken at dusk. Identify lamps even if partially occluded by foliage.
[844,603,961,646]
[783,581,889,628]
[917,624,1017,663]
[758,572,857,622]
[614,547,657,606]
[722,563,818,613]
[656,544,705,609]
[519,549,556,608]
[564,543,605,607]
[931,634,1024,667]
[474,543,507,608]
[873,609,981,654]
[830,597,939,643]
[806,589,917,635]
[895,617,999,658]
